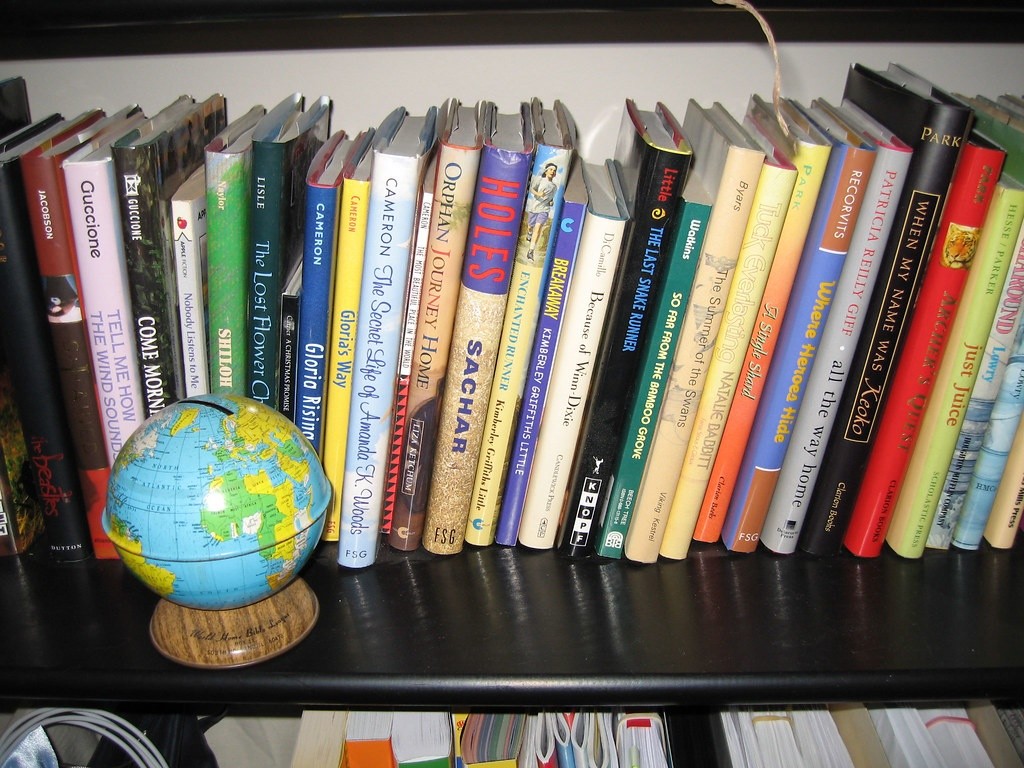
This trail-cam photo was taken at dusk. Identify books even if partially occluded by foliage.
[0,59,1023,566]
[0,707,1024,768]
